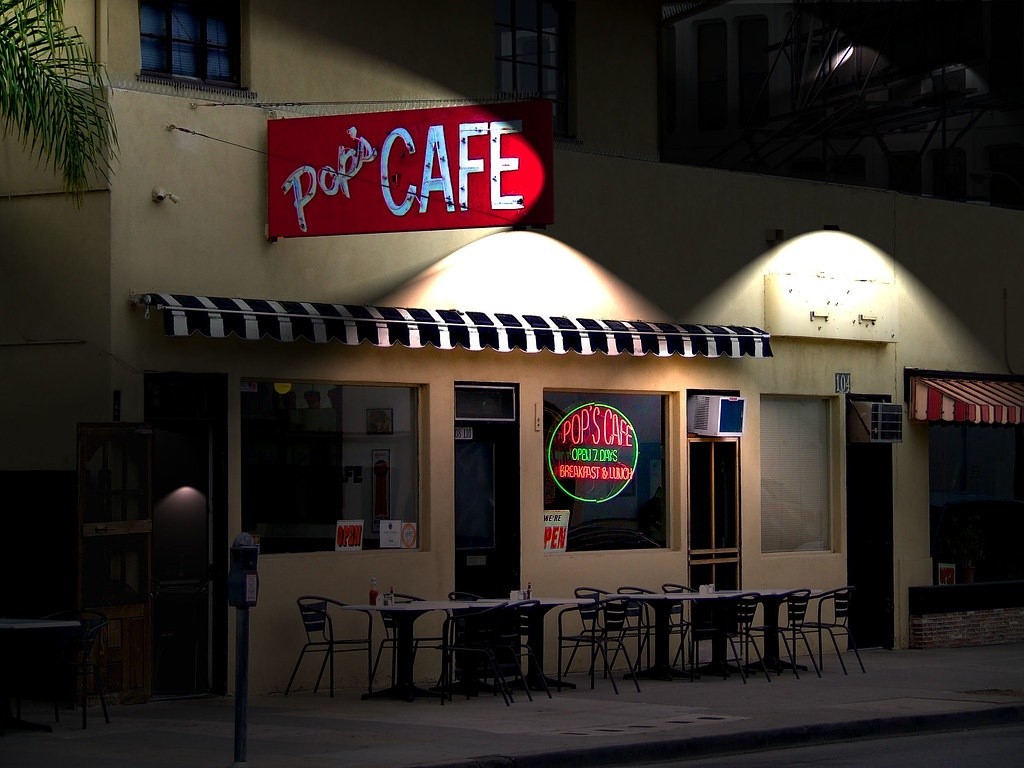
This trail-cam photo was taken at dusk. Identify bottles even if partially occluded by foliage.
[510,590,526,601]
[370,578,378,605]
[527,582,532,599]
[699,584,715,594]
[390,587,394,605]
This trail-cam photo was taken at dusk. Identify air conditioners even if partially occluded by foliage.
[847,399,902,443]
[689,394,748,437]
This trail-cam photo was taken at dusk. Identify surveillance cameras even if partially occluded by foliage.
[157,191,166,200]
[170,193,180,203]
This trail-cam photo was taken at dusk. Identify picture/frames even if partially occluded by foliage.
[365,407,394,435]
[371,449,390,533]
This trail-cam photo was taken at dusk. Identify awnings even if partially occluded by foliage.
[909,378,1024,425]
[138,292,773,359]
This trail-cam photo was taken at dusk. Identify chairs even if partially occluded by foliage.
[15,608,111,729]
[379,583,879,707]
[284,596,374,699]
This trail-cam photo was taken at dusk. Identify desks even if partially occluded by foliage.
[0,619,83,734]
[339,598,595,701]
[609,590,825,682]
